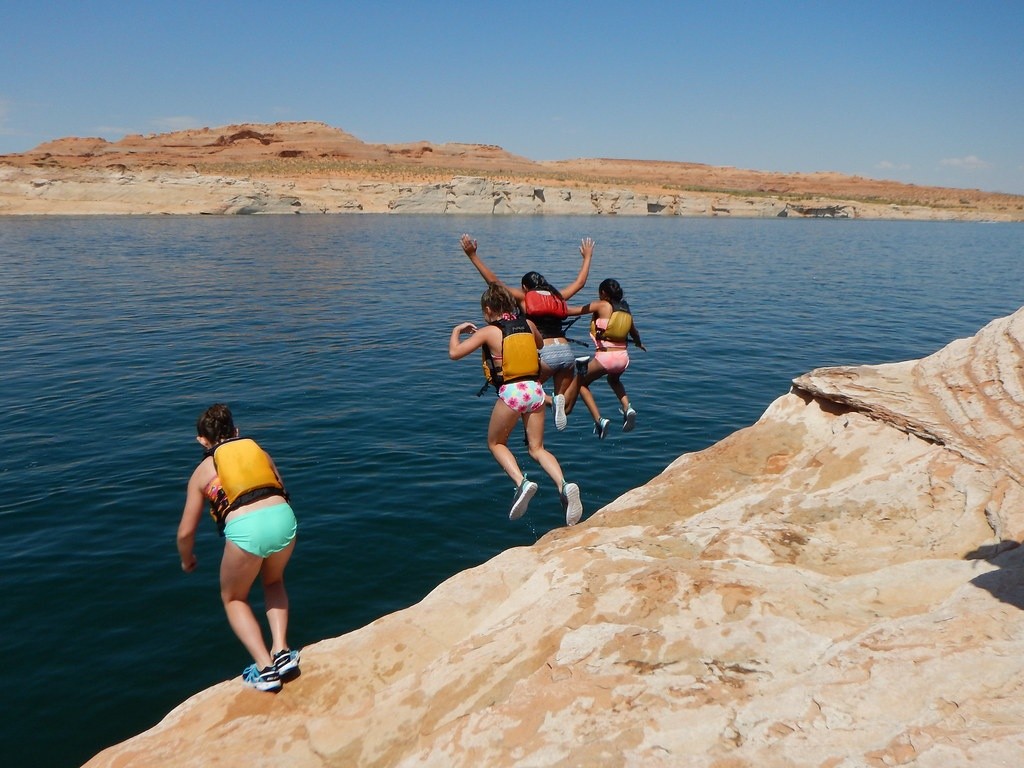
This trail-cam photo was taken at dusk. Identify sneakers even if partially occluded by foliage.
[550,392,567,431]
[243,663,282,691]
[593,417,610,440]
[508,473,538,520]
[569,356,590,377]
[272,649,299,676]
[560,479,583,526]
[619,403,635,432]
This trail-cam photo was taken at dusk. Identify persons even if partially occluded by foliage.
[566,278,646,439]
[177,403,300,691]
[449,282,583,526]
[460,233,595,432]
